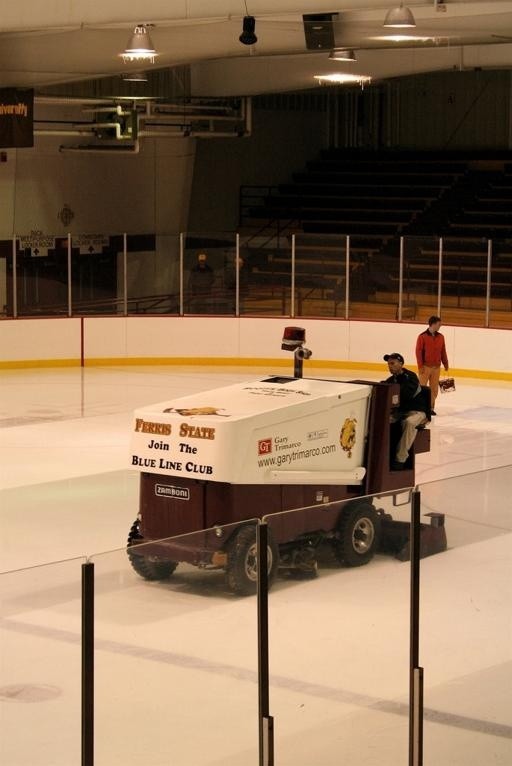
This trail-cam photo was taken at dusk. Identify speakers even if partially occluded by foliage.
[302,13,336,49]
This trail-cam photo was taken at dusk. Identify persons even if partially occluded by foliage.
[337,245,370,304]
[416,315,449,415]
[380,352,431,470]
[231,257,250,313]
[188,252,214,313]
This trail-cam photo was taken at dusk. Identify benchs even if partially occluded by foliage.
[247,150,512,288]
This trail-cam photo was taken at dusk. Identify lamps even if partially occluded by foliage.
[117,23,161,52]
[328,46,360,62]
[383,0,417,27]
[238,0,257,46]
[122,71,148,82]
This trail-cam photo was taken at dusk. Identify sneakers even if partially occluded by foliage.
[391,460,404,470]
[431,410,435,415]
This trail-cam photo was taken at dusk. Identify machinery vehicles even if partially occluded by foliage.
[126,326,447,595]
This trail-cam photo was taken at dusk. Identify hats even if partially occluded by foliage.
[382,352,404,365]
